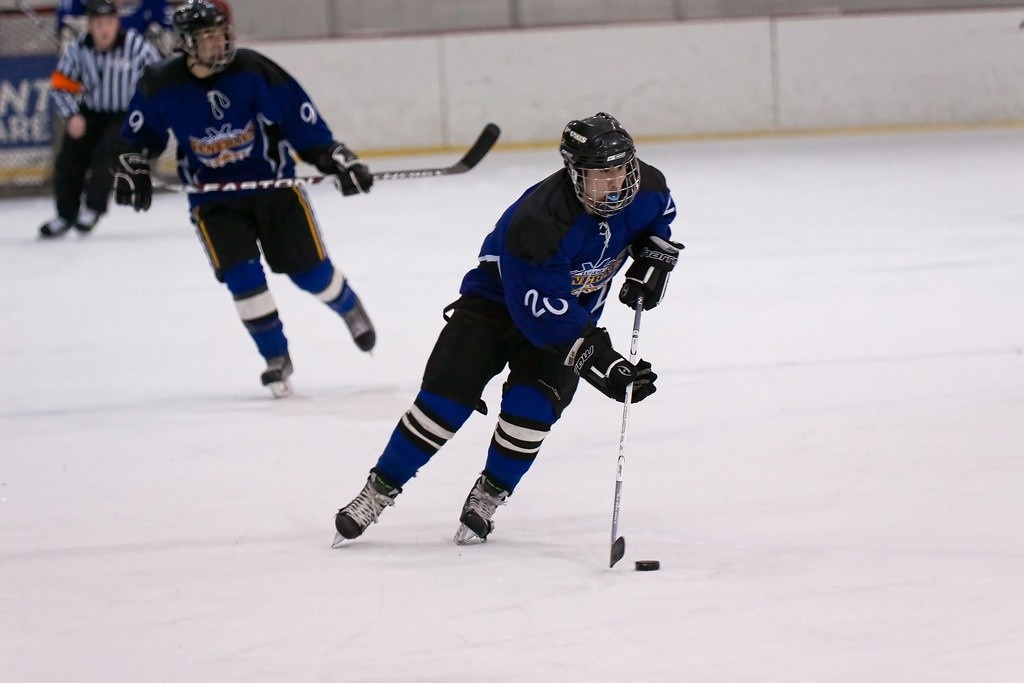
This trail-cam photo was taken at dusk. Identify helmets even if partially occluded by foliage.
[173,2,233,67]
[87,0,117,18]
[560,112,635,218]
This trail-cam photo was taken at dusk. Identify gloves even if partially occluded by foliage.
[113,148,152,211]
[619,236,686,311]
[319,142,374,197]
[566,328,658,404]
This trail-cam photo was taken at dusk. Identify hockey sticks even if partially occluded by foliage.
[155,122,503,195]
[610,290,645,568]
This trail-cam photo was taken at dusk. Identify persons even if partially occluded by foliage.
[113,1,376,398]
[38,0,164,237]
[331,112,686,548]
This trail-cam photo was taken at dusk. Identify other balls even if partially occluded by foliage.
[631,559,661,571]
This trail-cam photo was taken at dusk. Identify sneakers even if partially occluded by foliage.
[38,216,71,241]
[76,208,102,240]
[345,297,377,358]
[454,473,512,545]
[260,357,296,397]
[331,466,402,548]
